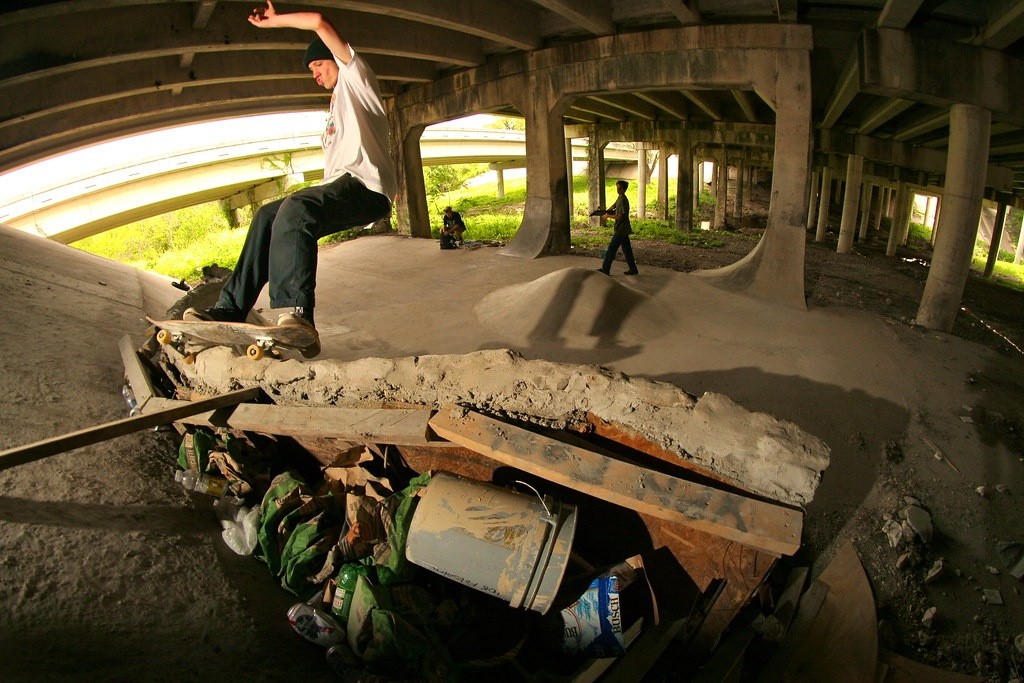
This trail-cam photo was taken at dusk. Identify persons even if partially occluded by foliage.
[596,181,638,276]
[440,206,466,245]
[183,0,397,333]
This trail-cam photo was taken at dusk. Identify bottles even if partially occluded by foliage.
[123,385,138,409]
[331,564,367,624]
[175,470,228,497]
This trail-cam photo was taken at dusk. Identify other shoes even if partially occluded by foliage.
[277,311,321,358]
[596,269,610,276]
[183,307,246,323]
[459,238,463,246]
[624,270,638,275]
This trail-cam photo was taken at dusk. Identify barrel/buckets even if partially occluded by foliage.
[406,471,577,616]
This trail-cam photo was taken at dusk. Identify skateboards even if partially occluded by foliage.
[589,206,616,216]
[144,315,319,361]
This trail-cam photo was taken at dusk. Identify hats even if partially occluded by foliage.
[443,206,452,211]
[303,39,334,71]
